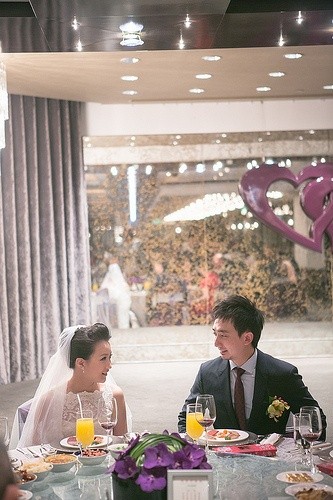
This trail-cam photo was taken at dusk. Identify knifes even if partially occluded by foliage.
[17,447,33,460]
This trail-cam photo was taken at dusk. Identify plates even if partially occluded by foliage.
[60,435,112,448]
[14,472,37,484]
[16,489,33,500]
[201,429,249,443]
[276,471,324,484]
[285,483,333,497]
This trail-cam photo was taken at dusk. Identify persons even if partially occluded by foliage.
[17,323,132,448]
[102,228,306,326]
[177,293,327,442]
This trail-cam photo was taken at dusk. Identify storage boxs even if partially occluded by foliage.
[212,444,277,459]
[316,460,333,477]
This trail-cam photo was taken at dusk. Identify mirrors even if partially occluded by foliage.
[81,128,333,329]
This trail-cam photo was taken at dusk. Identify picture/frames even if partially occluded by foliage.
[168,469,213,500]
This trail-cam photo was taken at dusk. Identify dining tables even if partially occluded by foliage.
[6,431,333,500]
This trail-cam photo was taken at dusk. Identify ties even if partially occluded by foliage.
[232,367,247,432]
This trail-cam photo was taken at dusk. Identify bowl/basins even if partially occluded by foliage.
[73,448,110,465]
[107,443,129,461]
[18,462,53,482]
[39,455,78,473]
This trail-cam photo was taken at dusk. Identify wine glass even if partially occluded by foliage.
[195,395,216,456]
[97,397,117,435]
[300,406,322,477]
[293,413,313,465]
[186,403,205,445]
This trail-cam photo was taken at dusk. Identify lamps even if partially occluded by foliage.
[119,31,143,47]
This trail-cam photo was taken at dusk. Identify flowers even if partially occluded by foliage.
[112,430,213,492]
[265,395,290,422]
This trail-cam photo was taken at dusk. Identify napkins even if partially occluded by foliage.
[264,432,281,447]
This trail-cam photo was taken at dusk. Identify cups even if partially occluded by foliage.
[0,417,9,446]
[76,418,94,448]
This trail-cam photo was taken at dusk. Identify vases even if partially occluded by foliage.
[111,472,167,500]
